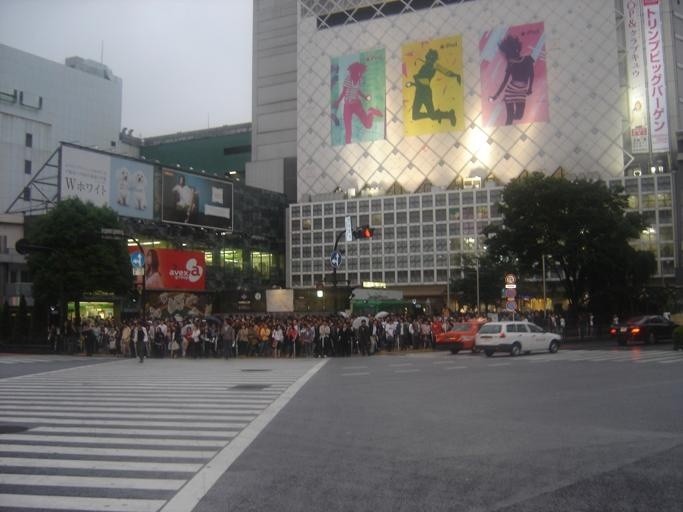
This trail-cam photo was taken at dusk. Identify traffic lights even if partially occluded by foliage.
[352,229,365,241]
[359,225,375,238]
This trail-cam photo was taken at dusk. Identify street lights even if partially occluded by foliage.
[448,240,452,308]
[541,254,553,318]
[475,252,481,308]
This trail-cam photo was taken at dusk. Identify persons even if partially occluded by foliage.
[144,250,163,288]
[412,49,460,126]
[499,313,567,344]
[173,175,197,213]
[333,62,382,145]
[608,312,623,324]
[45,311,488,357]
[487,35,534,125]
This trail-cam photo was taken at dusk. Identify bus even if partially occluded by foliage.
[350,297,428,319]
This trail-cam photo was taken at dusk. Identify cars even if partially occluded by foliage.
[476,322,562,355]
[610,313,680,346]
[669,326,682,350]
[434,320,487,353]
